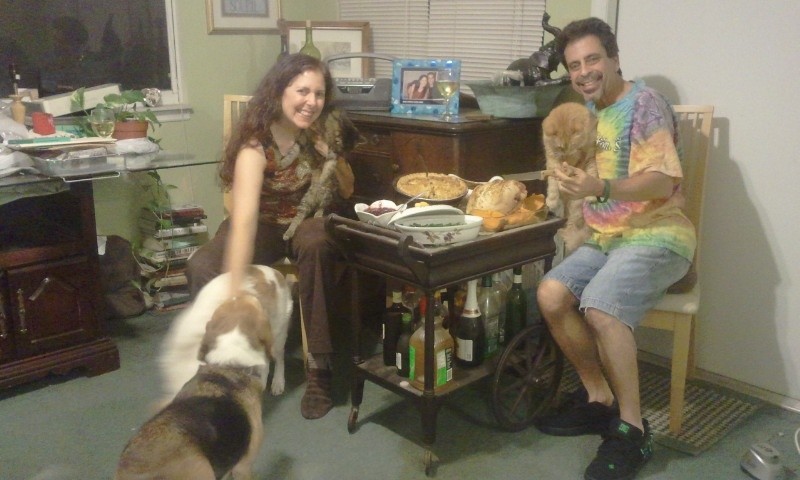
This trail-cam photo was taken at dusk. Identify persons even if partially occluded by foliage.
[532,15,698,480]
[409,75,430,99]
[183,53,356,420]
[410,72,440,99]
[33,16,111,92]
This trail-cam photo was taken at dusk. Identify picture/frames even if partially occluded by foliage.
[390,58,461,115]
[206,0,282,35]
[281,21,371,89]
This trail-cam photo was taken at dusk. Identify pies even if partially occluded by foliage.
[396,172,468,201]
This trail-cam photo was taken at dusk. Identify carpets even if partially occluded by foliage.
[507,350,763,457]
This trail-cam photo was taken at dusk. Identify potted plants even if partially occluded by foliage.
[70,87,178,303]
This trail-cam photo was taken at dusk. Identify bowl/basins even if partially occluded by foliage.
[354,199,406,228]
[390,202,483,247]
[393,171,468,208]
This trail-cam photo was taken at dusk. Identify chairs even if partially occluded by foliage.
[223,94,310,379]
[582,105,714,435]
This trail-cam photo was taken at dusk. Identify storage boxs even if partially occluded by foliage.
[23,84,122,117]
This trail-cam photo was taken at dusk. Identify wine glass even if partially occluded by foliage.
[90,108,116,158]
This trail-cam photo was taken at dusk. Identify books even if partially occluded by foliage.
[140,207,208,312]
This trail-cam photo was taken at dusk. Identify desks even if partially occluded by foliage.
[0,149,228,392]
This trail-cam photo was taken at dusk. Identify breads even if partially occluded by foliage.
[466,179,527,218]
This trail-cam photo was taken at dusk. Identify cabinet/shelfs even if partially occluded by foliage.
[0,255,97,366]
[337,108,546,333]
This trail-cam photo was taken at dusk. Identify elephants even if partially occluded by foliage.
[503,13,563,86]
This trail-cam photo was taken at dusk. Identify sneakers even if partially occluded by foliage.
[534,387,621,435]
[302,365,336,419]
[583,417,652,480]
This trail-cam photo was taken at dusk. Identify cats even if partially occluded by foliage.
[282,111,365,241]
[526,102,599,252]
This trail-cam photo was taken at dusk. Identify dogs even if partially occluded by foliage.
[115,291,274,480]
[154,265,292,412]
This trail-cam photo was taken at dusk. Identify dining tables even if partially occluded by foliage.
[323,213,565,477]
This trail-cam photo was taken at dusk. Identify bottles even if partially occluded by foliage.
[300,25,322,64]
[377,262,527,390]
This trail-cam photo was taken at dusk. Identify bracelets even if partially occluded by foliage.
[596,179,609,203]
